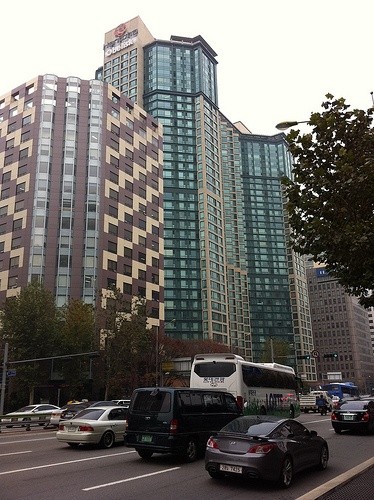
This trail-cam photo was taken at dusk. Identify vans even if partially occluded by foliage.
[123,387,247,462]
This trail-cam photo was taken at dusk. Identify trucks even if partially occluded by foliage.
[299,390,333,413]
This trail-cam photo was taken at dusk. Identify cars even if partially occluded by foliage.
[50,399,129,448]
[1,404,60,428]
[331,400,373,433]
[204,414,330,489]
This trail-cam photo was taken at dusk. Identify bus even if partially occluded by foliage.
[189,352,300,419]
[320,383,362,400]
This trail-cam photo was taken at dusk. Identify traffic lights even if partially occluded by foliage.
[297,354,310,360]
[323,352,338,358]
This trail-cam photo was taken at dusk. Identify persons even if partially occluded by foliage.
[316,395,329,413]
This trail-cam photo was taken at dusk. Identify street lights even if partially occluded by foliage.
[155,319,177,386]
